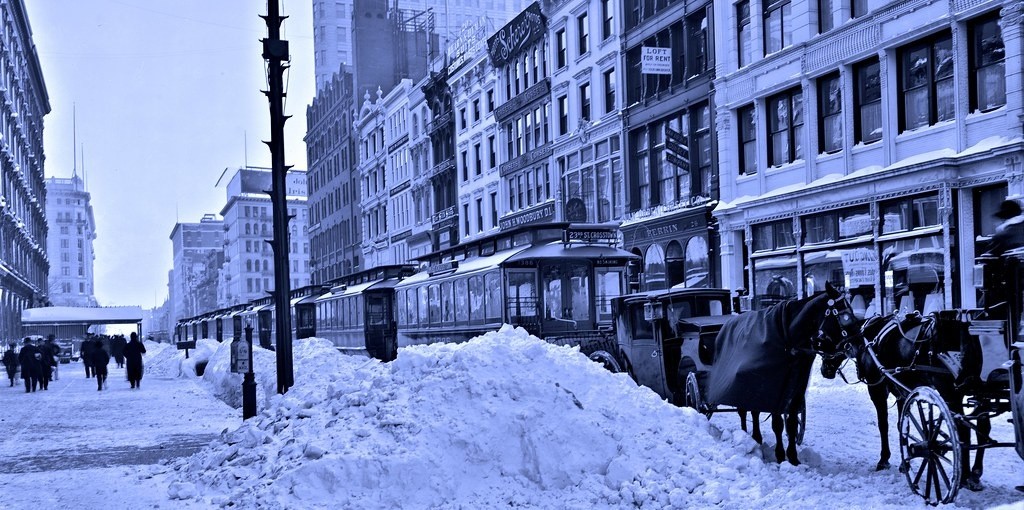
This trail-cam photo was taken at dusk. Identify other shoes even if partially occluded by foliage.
[131,385,135,388]
[10,383,13,387]
[40,387,43,390]
[25,389,30,393]
[44,387,47,390]
[137,386,140,388]
[32,387,36,392]
[97,388,102,391]
[104,387,108,390]
[121,366,124,368]
[86,375,90,378]
[117,366,119,368]
[92,374,95,377]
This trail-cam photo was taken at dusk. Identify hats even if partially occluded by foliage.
[131,332,138,337]
[991,200,1022,220]
[23,337,31,343]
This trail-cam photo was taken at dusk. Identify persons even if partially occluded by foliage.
[2,334,62,393]
[80,331,146,391]
[975,200,1024,317]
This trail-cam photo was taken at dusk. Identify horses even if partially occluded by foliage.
[736,280,991,493]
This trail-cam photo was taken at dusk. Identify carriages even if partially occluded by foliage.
[818,235,1024,505]
[609,288,866,468]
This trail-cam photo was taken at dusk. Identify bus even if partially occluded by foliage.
[396,220,642,356]
[147,331,172,344]
[173,282,332,351]
[314,264,419,363]
